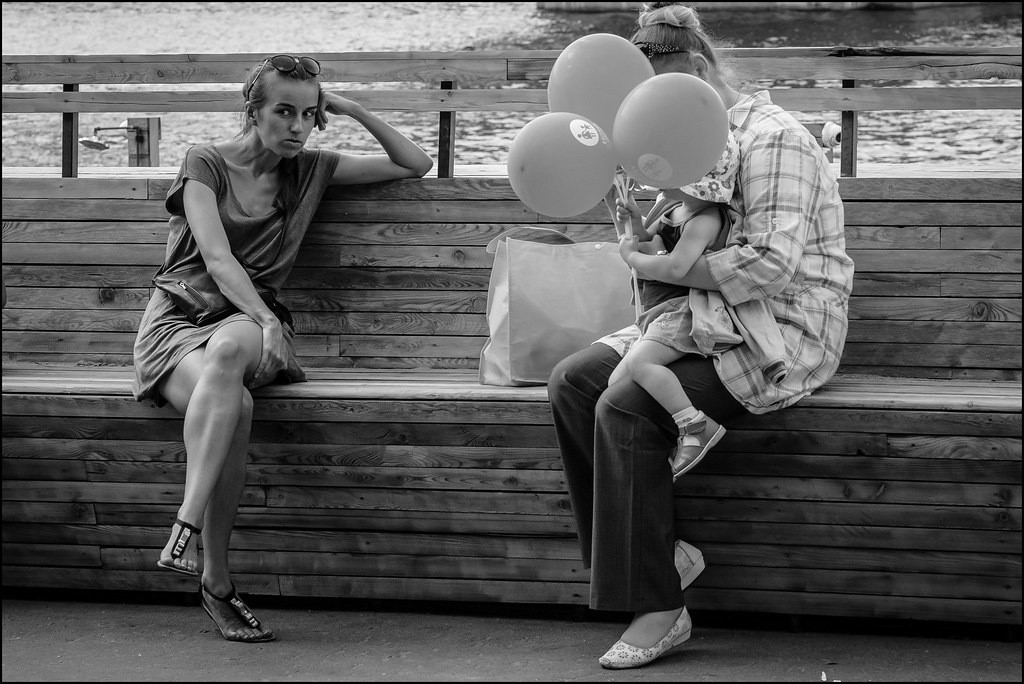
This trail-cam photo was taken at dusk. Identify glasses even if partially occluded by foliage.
[632,41,694,62]
[246,54,321,101]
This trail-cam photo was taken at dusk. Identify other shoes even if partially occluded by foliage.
[599,604,692,669]
[674,539,705,591]
[671,409,726,477]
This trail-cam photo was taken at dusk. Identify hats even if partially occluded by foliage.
[679,128,740,205]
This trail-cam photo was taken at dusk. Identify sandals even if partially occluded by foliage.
[157,518,201,575]
[198,577,275,642]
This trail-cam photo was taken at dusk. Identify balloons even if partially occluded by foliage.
[507,33,729,219]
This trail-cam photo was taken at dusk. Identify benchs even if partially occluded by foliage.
[2,174,1022,627]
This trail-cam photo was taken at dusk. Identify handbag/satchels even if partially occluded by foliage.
[479,227,645,387]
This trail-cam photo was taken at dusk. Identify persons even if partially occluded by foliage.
[131,55,434,643]
[547,3,854,671]
